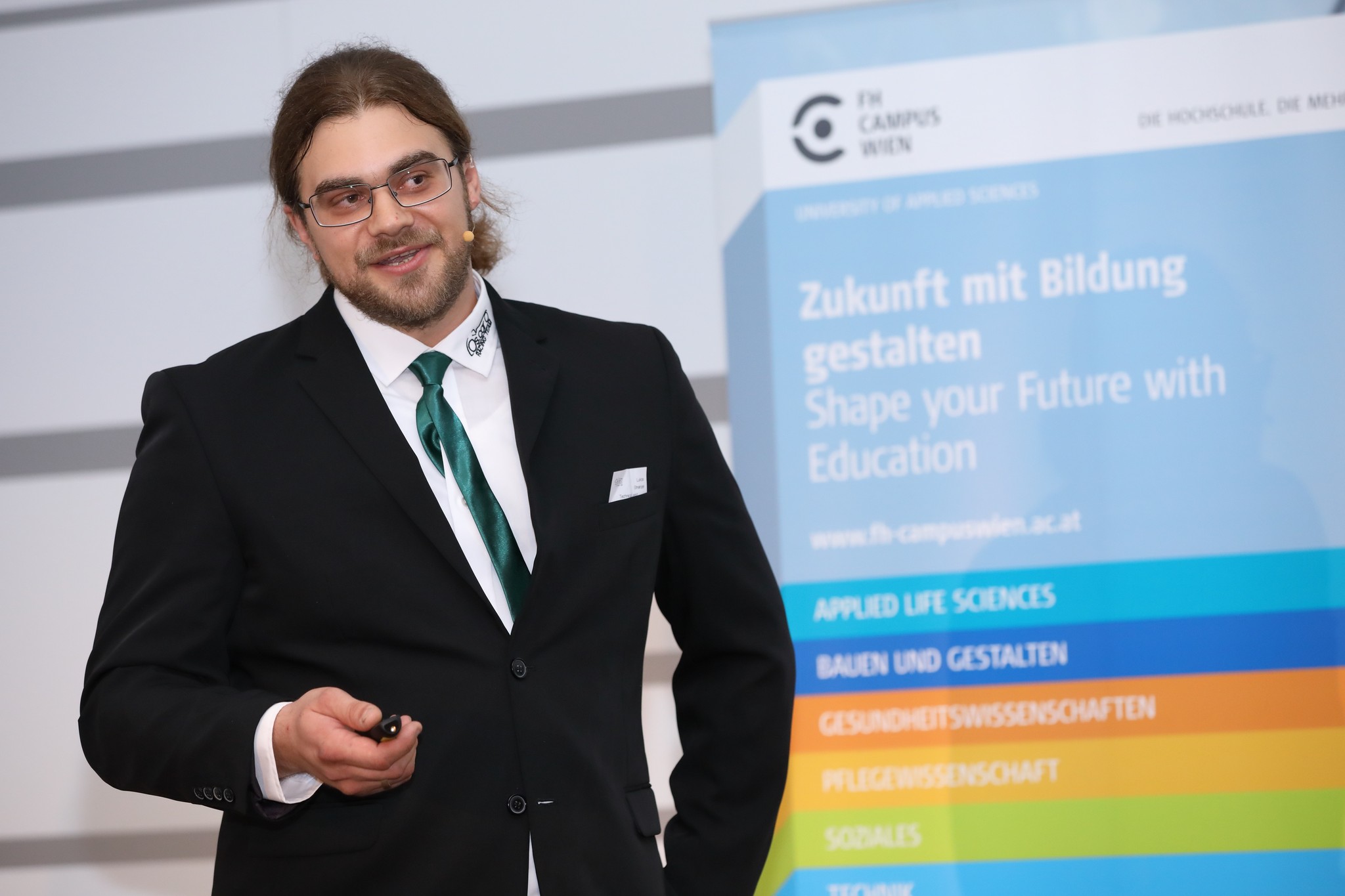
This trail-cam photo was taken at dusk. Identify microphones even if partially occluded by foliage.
[464,223,475,242]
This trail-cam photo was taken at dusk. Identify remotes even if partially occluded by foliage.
[366,715,403,743]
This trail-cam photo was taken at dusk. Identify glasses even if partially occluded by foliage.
[298,155,463,228]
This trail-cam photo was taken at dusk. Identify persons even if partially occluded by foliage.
[78,43,797,894]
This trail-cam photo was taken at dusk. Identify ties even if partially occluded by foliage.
[409,350,531,623]
